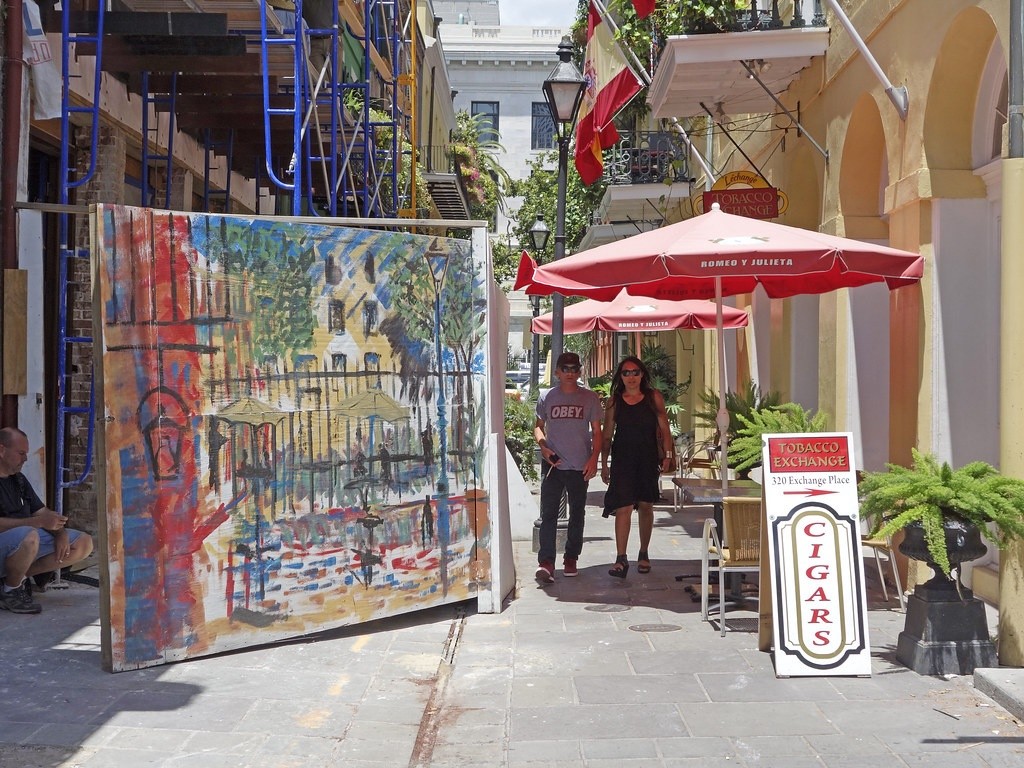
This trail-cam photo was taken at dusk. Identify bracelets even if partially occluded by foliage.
[664,451,672,459]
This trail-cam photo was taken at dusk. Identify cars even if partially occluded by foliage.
[504,367,587,405]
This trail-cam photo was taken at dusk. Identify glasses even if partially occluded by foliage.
[559,366,579,373]
[620,369,641,376]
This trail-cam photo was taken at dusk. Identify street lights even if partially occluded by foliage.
[528,213,552,404]
[533,33,596,557]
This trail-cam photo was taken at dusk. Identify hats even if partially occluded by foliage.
[557,353,582,367]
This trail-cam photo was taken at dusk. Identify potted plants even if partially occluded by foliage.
[858,446,1024,603]
[692,379,785,480]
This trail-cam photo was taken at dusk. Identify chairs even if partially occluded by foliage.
[702,496,761,637]
[855,470,906,614]
[658,429,721,512]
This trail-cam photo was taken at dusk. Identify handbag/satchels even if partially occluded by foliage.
[651,390,676,474]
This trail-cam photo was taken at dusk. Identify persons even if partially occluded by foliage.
[603,357,672,580]
[0,427,93,615]
[534,352,602,585]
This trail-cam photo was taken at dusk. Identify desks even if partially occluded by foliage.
[672,478,762,603]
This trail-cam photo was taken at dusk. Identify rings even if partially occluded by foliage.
[593,473,595,477]
[66,553,69,554]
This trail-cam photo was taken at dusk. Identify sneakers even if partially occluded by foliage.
[0,580,42,614]
[564,557,578,577]
[535,560,554,583]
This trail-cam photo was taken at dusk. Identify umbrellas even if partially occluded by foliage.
[514,202,923,490]
[530,288,747,335]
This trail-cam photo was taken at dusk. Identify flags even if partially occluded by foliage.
[631,0,655,19]
[574,0,643,188]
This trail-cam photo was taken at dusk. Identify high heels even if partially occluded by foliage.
[609,555,629,579]
[637,551,650,573]
